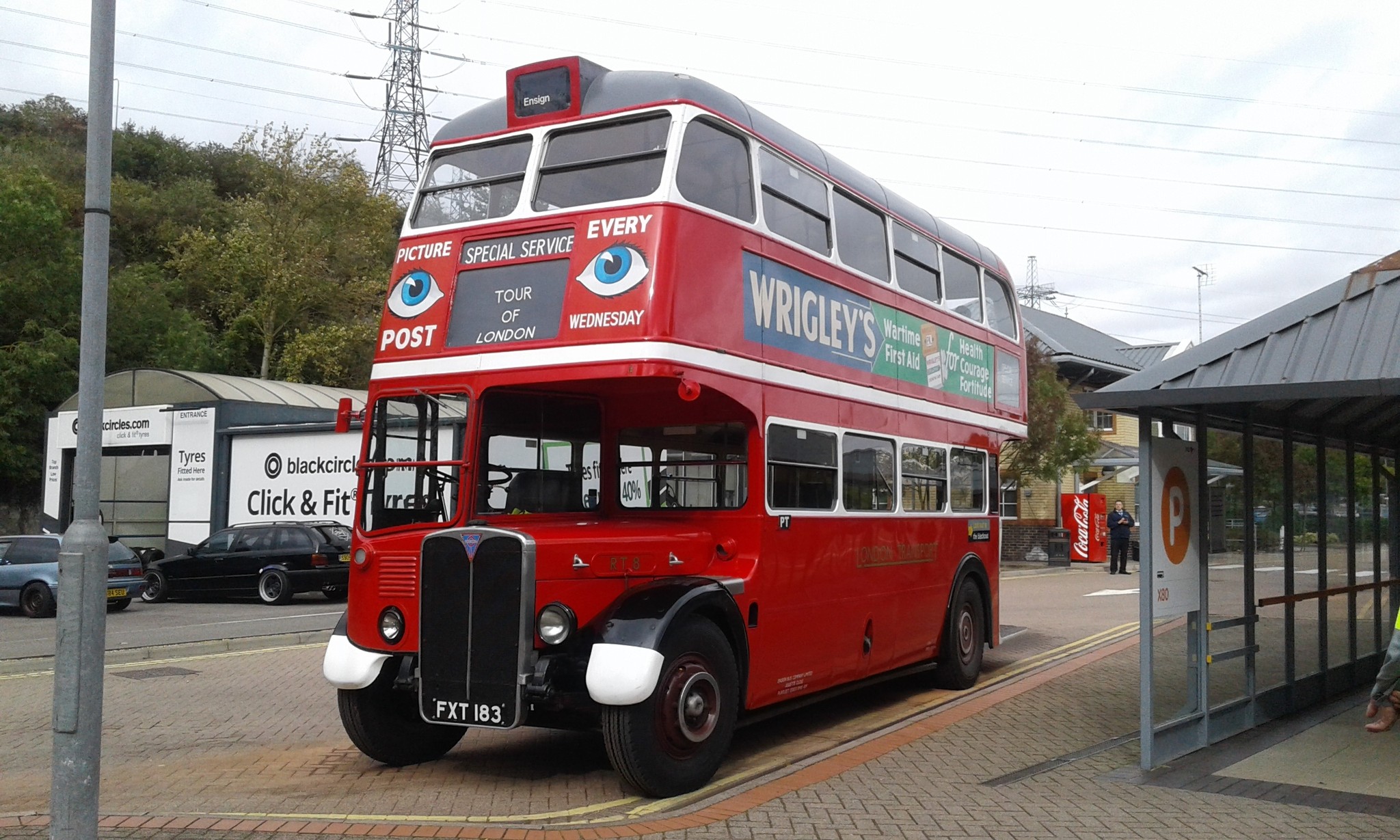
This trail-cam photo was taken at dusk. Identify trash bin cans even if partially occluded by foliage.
[1133,541,1140,562]
[1048,527,1071,567]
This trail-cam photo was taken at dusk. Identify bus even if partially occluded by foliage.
[324,53,1033,801]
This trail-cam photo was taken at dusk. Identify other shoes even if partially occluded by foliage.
[1110,571,1117,574]
[1364,711,1399,733]
[1365,698,1378,719]
[1118,571,1132,575]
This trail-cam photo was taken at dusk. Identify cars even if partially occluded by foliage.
[0,535,147,619]
[1240,502,1360,522]
[141,519,352,605]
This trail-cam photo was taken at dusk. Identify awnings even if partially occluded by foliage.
[1072,434,1244,493]
[1000,478,1018,491]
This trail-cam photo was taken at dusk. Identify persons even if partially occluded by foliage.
[1365,604,1400,732]
[1107,500,1135,575]
